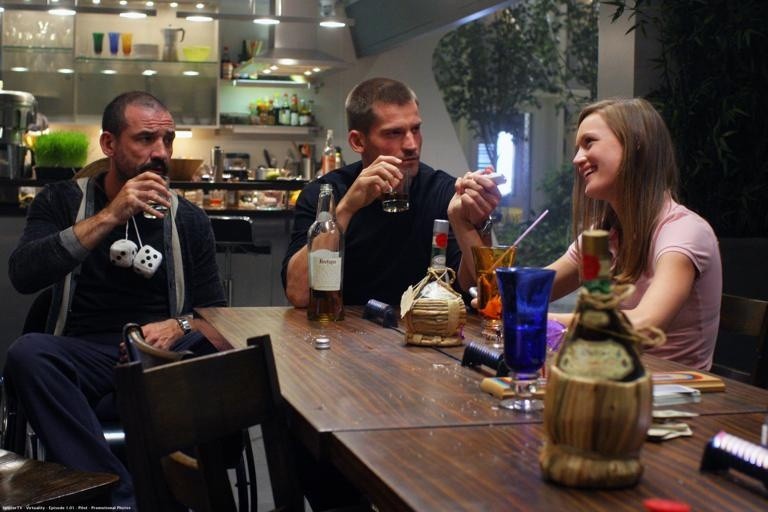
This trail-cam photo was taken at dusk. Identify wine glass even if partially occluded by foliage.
[470,245,518,350]
[495,267,557,413]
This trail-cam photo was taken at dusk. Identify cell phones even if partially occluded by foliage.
[481,173,507,185]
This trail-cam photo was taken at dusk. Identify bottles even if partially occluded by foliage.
[221,47,235,79]
[237,39,253,79]
[249,93,316,127]
[405,220,467,347]
[210,145,225,183]
[538,224,655,488]
[321,129,343,177]
[305,183,346,323]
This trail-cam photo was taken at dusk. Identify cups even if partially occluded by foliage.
[381,168,411,214]
[92,32,132,58]
[142,173,171,220]
[209,190,226,207]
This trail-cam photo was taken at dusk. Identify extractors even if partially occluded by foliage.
[233,0,349,78]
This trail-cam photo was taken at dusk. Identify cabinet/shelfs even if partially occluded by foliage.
[224,78,321,131]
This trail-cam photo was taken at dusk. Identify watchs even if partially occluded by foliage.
[478,214,493,235]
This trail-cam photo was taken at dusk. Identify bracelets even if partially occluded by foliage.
[175,316,191,335]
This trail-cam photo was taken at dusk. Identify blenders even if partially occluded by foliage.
[0,89,38,179]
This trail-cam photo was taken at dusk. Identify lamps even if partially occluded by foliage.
[177,11,356,28]
[0,3,156,19]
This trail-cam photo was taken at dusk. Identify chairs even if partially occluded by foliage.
[208,215,257,307]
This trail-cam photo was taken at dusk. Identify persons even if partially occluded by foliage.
[109,230,165,278]
[447,97,723,370]
[281,78,495,309]
[3,90,229,511]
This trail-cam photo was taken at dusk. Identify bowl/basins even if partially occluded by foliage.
[133,42,160,59]
[167,159,205,183]
[183,46,212,62]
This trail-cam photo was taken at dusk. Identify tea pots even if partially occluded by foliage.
[159,23,185,61]
